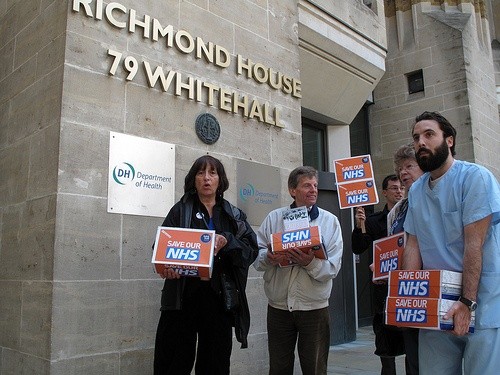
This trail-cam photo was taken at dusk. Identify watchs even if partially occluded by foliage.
[459,295,479,312]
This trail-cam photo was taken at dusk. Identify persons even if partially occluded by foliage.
[251,166,343,375]
[369,141,424,375]
[351,173,407,285]
[151,156,259,375]
[400,111,500,375]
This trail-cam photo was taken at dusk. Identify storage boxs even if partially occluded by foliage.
[385,297,476,334]
[385,269,475,299]
[270,226,328,268]
[151,226,215,279]
[373,232,408,285]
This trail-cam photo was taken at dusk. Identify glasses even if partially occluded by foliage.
[386,187,405,191]
[398,164,418,171]
